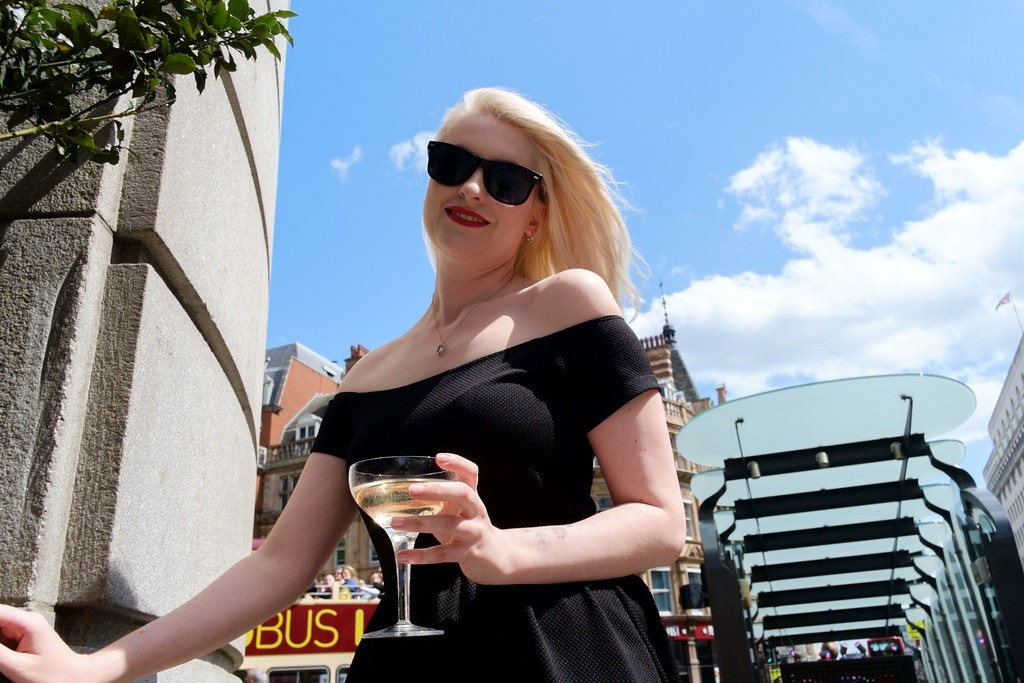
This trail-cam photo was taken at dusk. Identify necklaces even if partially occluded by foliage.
[432,290,495,356]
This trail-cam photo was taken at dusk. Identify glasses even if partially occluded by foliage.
[427,140,546,206]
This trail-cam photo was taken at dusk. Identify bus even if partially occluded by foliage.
[232,583,382,683]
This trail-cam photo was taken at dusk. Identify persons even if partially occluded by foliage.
[299,566,384,601]
[0,87,687,683]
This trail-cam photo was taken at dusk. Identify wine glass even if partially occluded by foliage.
[349,455,458,638]
